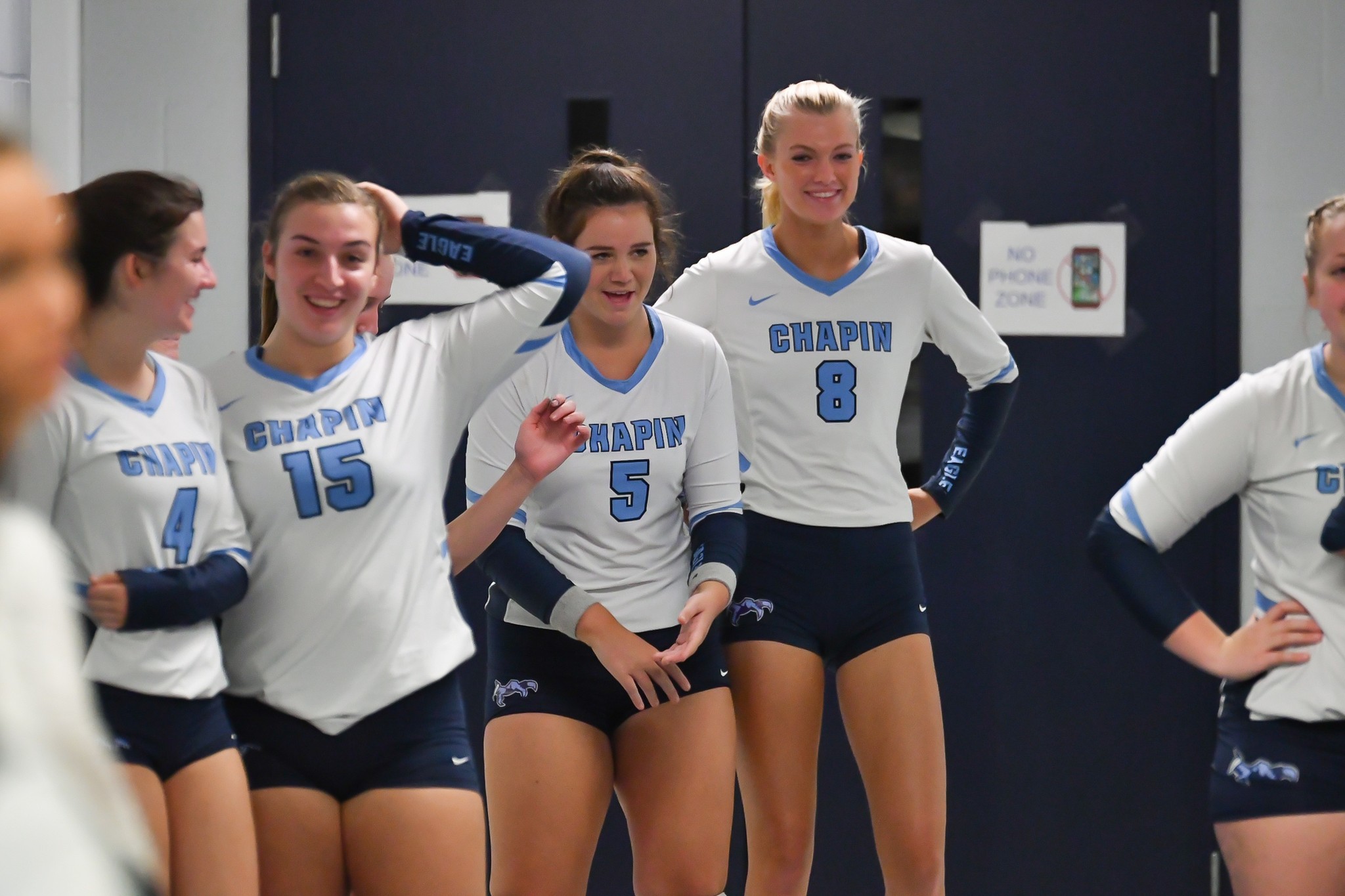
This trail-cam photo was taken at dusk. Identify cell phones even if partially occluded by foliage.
[1071,248,1101,308]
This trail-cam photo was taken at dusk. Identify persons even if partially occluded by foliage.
[0,126,261,896]
[1091,194,1345,896]
[653,79,1022,896]
[353,247,591,573]
[201,173,592,896]
[465,145,744,896]
